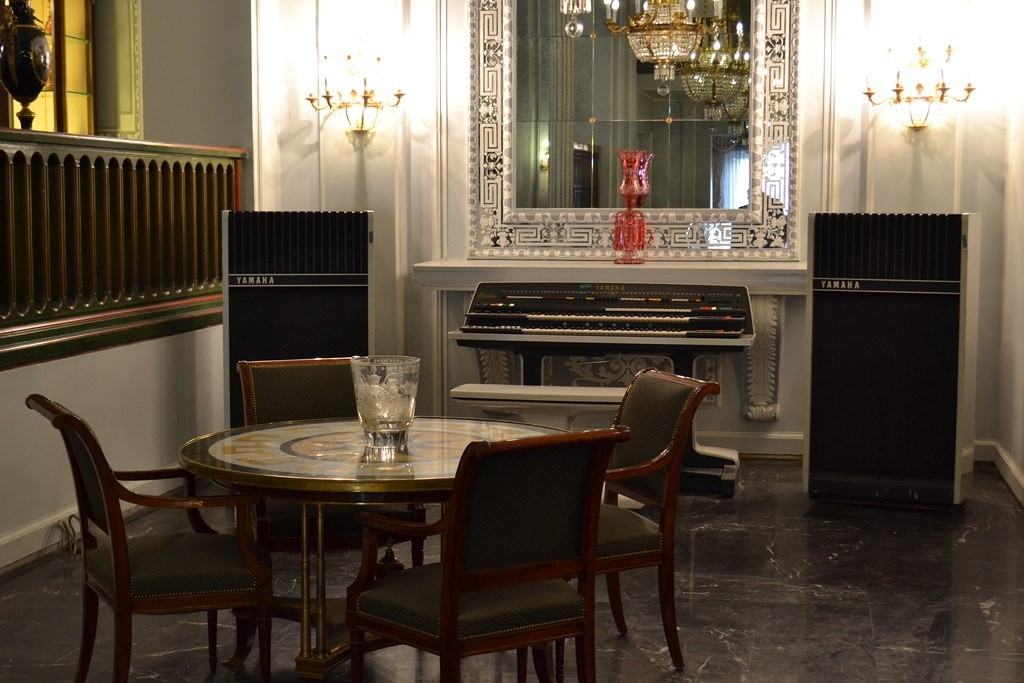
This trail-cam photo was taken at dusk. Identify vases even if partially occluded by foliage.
[350,356,420,451]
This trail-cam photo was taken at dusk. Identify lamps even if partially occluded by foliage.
[305,50,406,135]
[559,0,751,132]
[862,39,978,131]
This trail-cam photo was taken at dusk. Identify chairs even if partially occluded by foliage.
[345,424,631,683]
[515,369,721,683]
[221,358,425,670]
[26,393,274,683]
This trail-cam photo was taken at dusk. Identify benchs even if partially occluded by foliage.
[452,382,628,431]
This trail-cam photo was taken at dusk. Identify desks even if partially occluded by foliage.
[179,415,571,683]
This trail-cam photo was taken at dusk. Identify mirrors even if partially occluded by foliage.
[470,1,800,261]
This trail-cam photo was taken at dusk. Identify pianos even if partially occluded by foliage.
[449,283,758,353]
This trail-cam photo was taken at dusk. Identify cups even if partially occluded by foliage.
[351,355,420,449]
